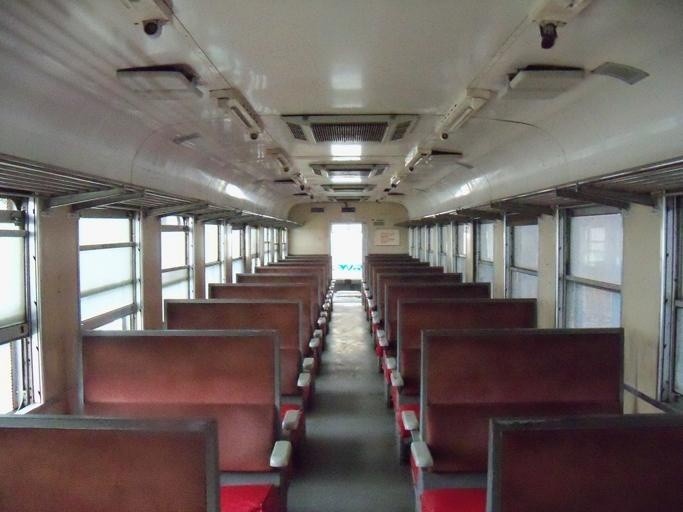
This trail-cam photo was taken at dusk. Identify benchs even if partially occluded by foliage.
[400,324,626,512]
[209,253,336,374]
[485,411,683,512]
[166,297,318,411]
[76,328,311,511]
[362,252,493,410]
[1,414,223,512]
[389,297,539,460]
